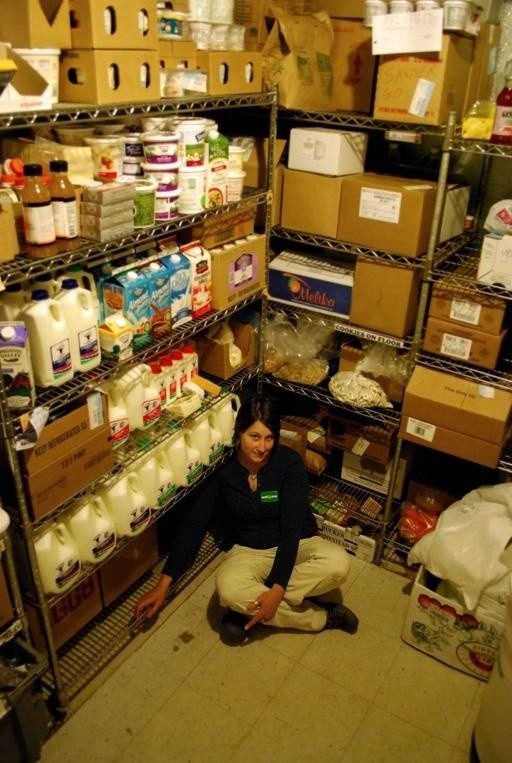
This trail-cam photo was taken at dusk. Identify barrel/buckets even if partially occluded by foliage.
[33,521,82,595]
[52,279,103,373]
[160,418,204,488]
[208,387,242,448]
[55,491,116,564]
[188,404,225,466]
[0,276,31,324]
[31,270,60,298]
[97,461,152,537]
[59,259,99,297]
[124,370,162,431]
[108,397,129,450]
[20,288,74,389]
[129,435,176,510]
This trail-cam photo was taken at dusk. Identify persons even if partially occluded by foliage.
[133,399,359,635]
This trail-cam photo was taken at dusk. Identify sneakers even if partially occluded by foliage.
[309,596,359,635]
[219,606,252,645]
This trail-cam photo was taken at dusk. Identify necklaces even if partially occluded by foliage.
[239,455,268,480]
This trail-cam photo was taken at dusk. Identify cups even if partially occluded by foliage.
[212,0,234,23]
[444,1,470,30]
[212,24,230,51]
[189,24,211,50]
[188,0,212,22]
[416,0,441,10]
[363,1,388,28]
[230,25,246,52]
[389,1,413,13]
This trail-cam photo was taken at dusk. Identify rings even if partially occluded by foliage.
[254,601,259,607]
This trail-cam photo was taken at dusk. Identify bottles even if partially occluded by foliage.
[491,76,512,144]
[46,161,78,240]
[21,164,56,245]
